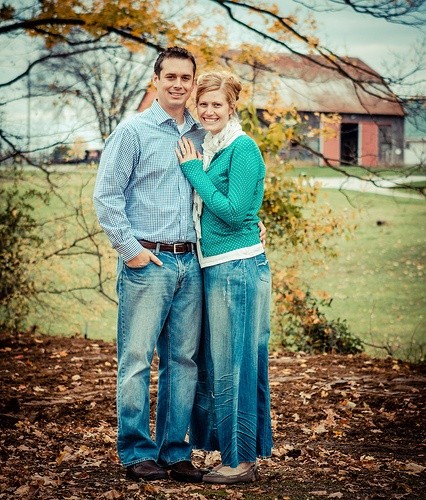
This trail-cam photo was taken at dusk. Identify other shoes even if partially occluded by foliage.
[203,462,261,487]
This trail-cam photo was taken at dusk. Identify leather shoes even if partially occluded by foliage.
[162,457,204,483]
[127,458,170,484]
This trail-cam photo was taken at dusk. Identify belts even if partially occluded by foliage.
[131,238,197,256]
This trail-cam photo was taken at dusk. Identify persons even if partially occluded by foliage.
[94,47,269,483]
[173,72,275,483]
